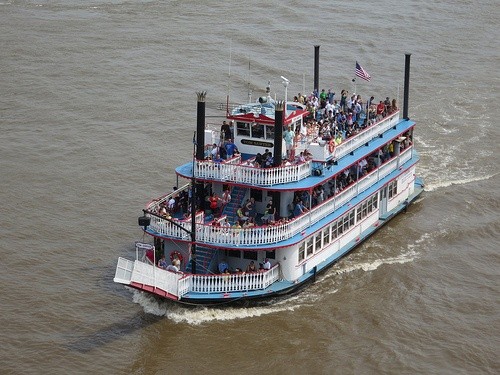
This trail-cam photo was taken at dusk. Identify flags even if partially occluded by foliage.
[355,62,370,83]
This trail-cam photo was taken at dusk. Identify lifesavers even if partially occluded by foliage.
[329,141,335,152]
[169,250,182,264]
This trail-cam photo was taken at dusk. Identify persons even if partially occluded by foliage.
[202,120,291,176]
[283,125,313,169]
[291,86,397,152]
[292,131,413,217]
[151,185,276,236]
[144,245,271,281]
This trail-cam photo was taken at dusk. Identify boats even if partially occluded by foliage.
[112,42,427,309]
